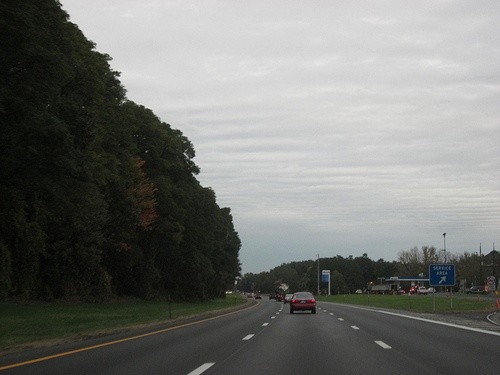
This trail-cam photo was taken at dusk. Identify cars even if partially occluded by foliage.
[465,286,486,294]
[254,292,293,303]
[357,284,436,295]
[290,291,317,315]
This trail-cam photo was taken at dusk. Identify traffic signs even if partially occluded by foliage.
[429,262,456,286]
